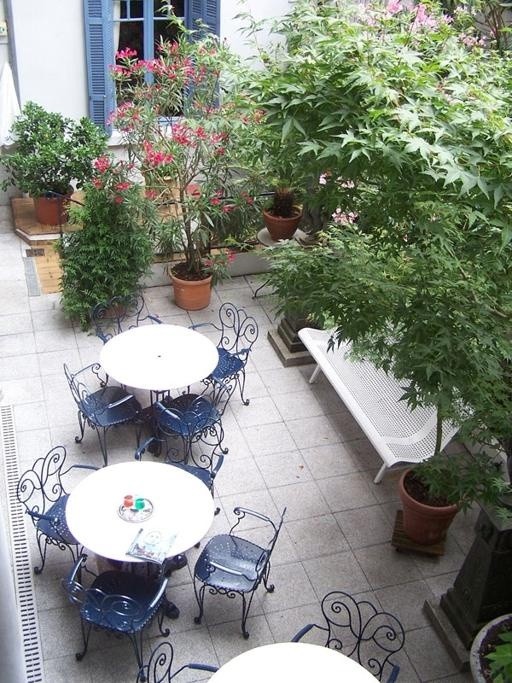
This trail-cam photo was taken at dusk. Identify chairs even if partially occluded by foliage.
[62,554,170,683]
[147,372,232,457]
[17,445,102,575]
[63,363,144,470]
[193,507,287,640]
[135,642,219,683]
[92,295,163,345]
[134,408,224,549]
[188,302,259,416]
[291,591,405,683]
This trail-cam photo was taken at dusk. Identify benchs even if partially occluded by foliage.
[297,306,477,484]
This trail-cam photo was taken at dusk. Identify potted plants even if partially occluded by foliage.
[0,101,112,225]
[468,613,512,683]
[265,187,303,242]
[265,223,512,545]
[52,180,156,332]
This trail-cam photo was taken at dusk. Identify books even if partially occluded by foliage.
[126,527,176,565]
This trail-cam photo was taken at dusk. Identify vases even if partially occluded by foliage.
[169,261,213,311]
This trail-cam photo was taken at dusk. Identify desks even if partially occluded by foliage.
[65,460,215,620]
[99,324,219,453]
[208,642,379,683]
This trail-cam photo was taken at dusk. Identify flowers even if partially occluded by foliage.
[94,0,264,283]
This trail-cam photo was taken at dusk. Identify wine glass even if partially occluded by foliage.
[135,498,145,516]
[123,495,134,516]
